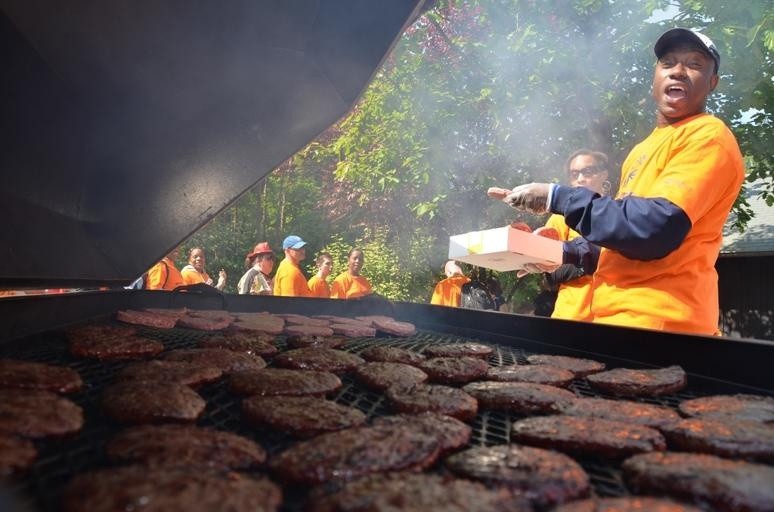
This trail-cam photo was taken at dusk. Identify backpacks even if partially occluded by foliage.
[461,278,496,313]
[131,260,170,290]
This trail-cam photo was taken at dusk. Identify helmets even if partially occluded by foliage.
[283,235,309,251]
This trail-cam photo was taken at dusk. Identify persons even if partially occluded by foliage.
[145,246,227,292]
[238,235,372,299]
[502,28,744,334]
[431,261,470,308]
[547,149,608,323]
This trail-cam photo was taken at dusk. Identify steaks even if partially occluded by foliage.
[0,305,774,512]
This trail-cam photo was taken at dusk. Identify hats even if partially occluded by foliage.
[653,27,721,70]
[246,241,274,258]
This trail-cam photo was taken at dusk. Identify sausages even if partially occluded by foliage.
[510,222,562,241]
[486,186,512,200]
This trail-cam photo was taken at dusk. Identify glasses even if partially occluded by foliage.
[568,163,608,182]
